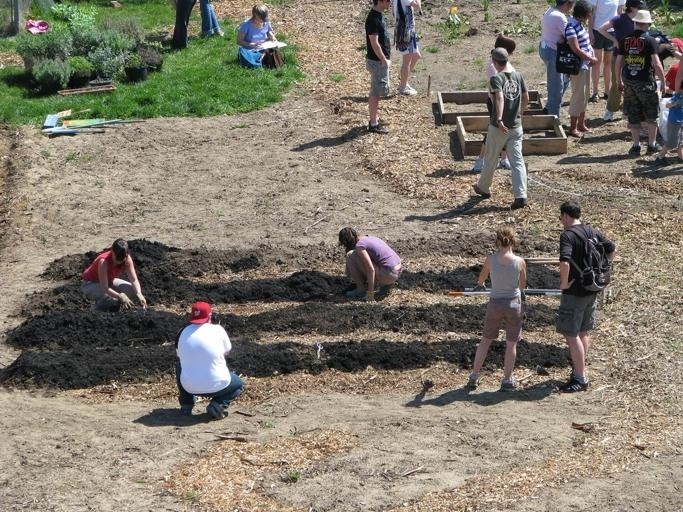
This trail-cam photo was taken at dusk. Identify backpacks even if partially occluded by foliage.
[561,226,610,293]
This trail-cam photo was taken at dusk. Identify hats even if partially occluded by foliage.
[632,10,654,24]
[672,37,683,53]
[491,47,509,61]
[187,302,211,324]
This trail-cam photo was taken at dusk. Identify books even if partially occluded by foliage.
[257,40,287,51]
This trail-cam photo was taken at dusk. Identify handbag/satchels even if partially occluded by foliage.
[393,15,410,48]
[555,43,582,75]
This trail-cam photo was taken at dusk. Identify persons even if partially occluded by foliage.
[175,301,245,420]
[559,201,615,393]
[473,33,516,172]
[539,0,683,163]
[80,237,147,312]
[200,0,224,37]
[473,47,529,209]
[339,226,403,302]
[469,227,527,391]
[393,0,421,95]
[365,0,392,130]
[237,4,284,69]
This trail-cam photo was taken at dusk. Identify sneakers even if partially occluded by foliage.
[346,283,393,297]
[181,402,225,419]
[469,373,587,393]
[570,93,682,166]
[369,84,527,209]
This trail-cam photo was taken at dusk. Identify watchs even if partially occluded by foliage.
[497,120,502,123]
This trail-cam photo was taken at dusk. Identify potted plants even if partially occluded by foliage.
[14,3,169,94]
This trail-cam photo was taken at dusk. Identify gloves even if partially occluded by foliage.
[118,292,146,311]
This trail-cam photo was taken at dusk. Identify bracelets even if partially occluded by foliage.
[367,291,375,297]
